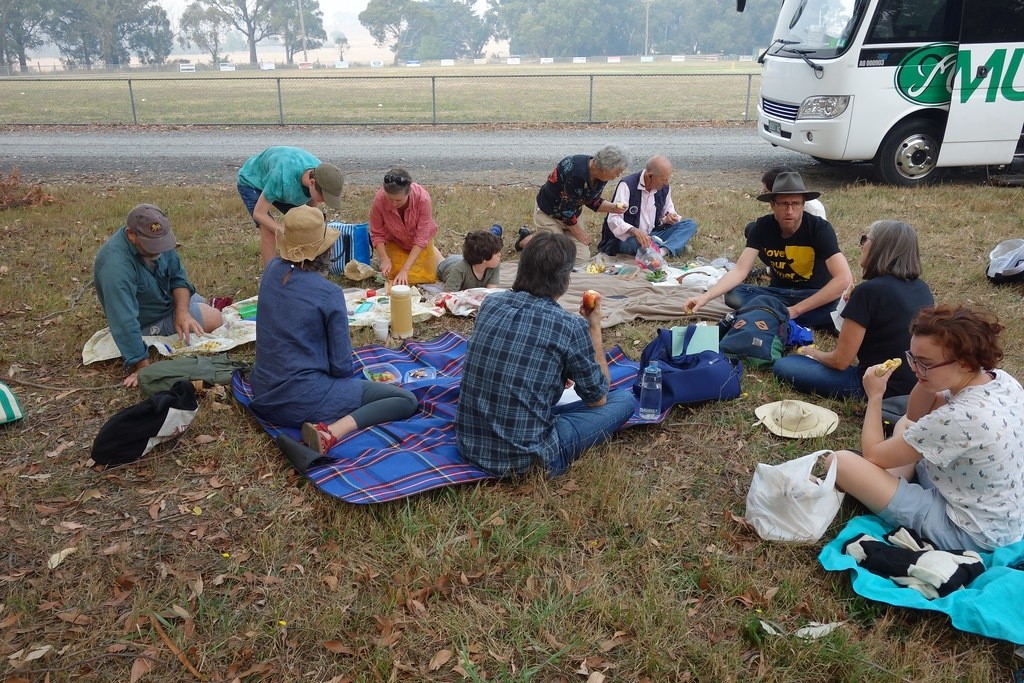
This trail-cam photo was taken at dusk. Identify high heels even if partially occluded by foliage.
[515,226,533,252]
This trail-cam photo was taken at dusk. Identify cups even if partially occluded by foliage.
[372,322,388,340]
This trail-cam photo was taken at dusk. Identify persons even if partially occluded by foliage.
[457,233,636,476]
[252,205,418,454]
[433,224,503,291]
[369,169,438,286]
[682,172,852,333]
[745,166,826,239]
[93,203,222,388]
[774,220,934,403]
[515,147,630,259]
[599,156,699,256]
[237,146,343,266]
[825,307,1024,553]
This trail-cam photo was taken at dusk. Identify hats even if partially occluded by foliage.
[755,399,839,439]
[126,204,177,253]
[756,172,820,203]
[313,163,344,210]
[275,205,341,263]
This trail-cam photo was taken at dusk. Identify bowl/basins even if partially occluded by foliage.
[363,362,402,386]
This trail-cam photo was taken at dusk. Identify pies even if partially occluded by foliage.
[875,358,902,377]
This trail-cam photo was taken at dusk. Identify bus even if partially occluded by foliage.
[756,0,1024,187]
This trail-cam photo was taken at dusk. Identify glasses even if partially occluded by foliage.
[773,199,804,211]
[905,348,964,378]
[383,174,412,187]
[859,233,874,246]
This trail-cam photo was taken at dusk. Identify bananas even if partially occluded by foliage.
[587,258,606,274]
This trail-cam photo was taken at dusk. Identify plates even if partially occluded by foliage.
[191,338,236,352]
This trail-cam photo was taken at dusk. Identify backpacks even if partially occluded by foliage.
[718,294,791,369]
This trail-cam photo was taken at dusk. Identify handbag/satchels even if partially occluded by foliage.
[323,219,374,275]
[633,326,741,408]
[89,379,199,466]
[746,449,845,547]
[135,348,257,396]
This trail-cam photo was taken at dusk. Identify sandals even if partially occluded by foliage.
[301,422,335,456]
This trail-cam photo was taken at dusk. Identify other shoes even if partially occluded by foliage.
[491,224,502,239]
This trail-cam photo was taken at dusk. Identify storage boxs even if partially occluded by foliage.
[404,366,436,385]
[362,361,402,387]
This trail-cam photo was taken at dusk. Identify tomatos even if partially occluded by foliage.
[652,260,660,266]
[367,290,376,298]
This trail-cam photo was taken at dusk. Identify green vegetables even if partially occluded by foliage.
[646,267,667,282]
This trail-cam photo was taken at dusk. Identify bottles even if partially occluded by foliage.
[390,285,413,339]
[640,361,662,420]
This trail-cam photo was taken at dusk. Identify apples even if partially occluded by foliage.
[583,290,600,309]
